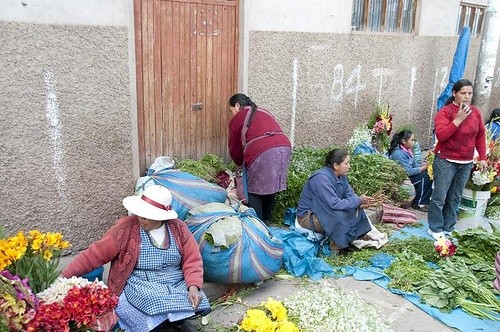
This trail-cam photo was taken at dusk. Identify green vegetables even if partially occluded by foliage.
[319,223,500,322]
[174,154,238,183]
[270,145,410,224]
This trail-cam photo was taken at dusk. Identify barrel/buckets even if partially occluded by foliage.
[456,188,491,218]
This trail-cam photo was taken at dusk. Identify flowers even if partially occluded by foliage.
[369,104,394,154]
[435,237,456,258]
[426,116,500,208]
[235,297,300,332]
[0,228,120,332]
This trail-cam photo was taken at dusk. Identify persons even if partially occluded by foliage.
[57,186,212,332]
[354,136,387,156]
[228,93,291,224]
[485,109,500,142]
[296,149,375,255]
[389,130,434,212]
[428,80,488,242]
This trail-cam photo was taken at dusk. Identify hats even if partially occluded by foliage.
[122,185,178,221]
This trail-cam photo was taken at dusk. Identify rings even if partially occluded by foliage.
[483,166,487,168]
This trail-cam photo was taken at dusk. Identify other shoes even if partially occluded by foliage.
[412,203,428,212]
[428,228,445,241]
[444,231,452,236]
